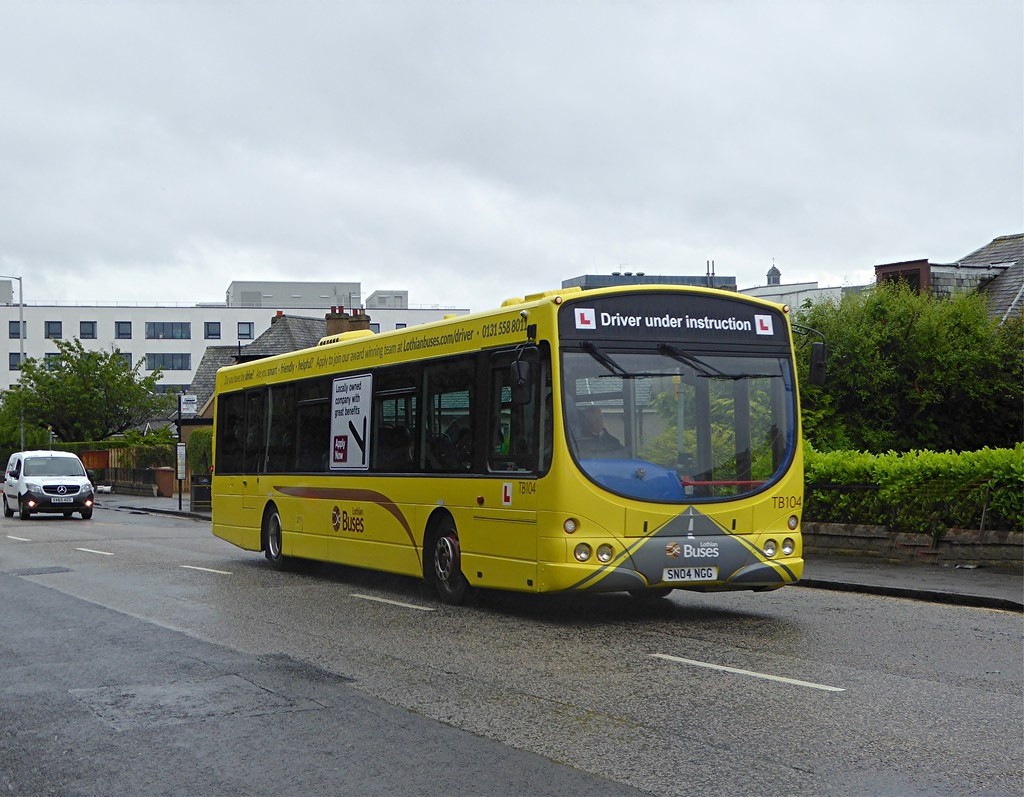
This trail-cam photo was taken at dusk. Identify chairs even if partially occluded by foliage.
[282,421,451,473]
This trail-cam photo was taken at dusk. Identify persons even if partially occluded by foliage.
[544,395,580,443]
[577,406,629,460]
[378,415,505,473]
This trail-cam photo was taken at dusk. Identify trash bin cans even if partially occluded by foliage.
[153,466,174,498]
[190,475,212,512]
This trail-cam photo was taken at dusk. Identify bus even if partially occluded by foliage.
[210,284,828,605]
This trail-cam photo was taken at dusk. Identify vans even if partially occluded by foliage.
[2,450,96,520]
